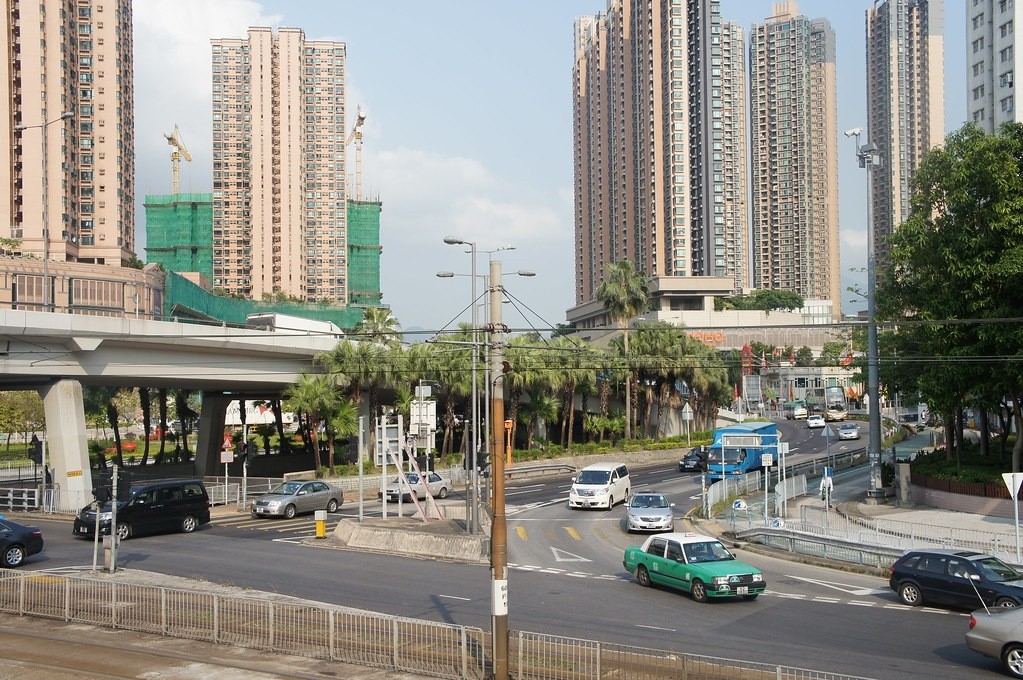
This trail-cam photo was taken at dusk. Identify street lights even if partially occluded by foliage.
[435,233,536,540]
[14,111,73,310]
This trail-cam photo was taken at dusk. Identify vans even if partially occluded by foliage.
[72,478,211,542]
[569,462,631,512]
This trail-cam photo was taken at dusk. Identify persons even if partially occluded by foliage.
[410,476,417,483]
[164,488,181,501]
[921,410,926,424]
[155,425,179,444]
[586,474,592,483]
[954,564,969,579]
[634,496,663,507]
[820,475,833,508]
[735,450,746,462]
[134,492,153,504]
[696,546,708,561]
[298,487,307,494]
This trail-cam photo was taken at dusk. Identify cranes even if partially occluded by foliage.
[346,105,366,203]
[165,123,192,202]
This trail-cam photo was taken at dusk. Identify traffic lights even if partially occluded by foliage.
[477,451,491,472]
[414,455,426,468]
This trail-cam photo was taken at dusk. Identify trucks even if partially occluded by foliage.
[706,421,778,484]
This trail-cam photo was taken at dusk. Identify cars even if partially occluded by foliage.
[0,519,43,568]
[965,606,1023,679]
[679,447,707,472]
[889,548,1023,608]
[250,480,344,519]
[623,491,676,533]
[805,414,826,428]
[378,471,452,503]
[622,532,766,602]
[837,422,861,440]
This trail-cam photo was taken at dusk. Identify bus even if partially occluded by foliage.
[782,385,848,422]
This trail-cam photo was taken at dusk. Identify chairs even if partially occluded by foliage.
[948,564,954,576]
